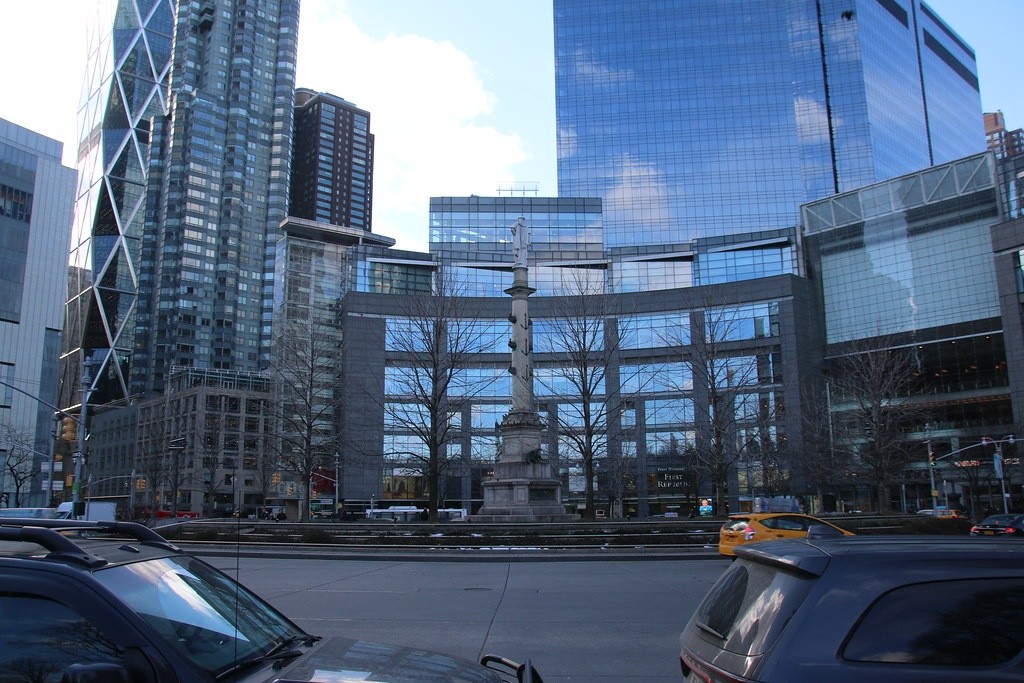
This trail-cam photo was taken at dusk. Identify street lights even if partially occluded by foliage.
[981,434,1015,513]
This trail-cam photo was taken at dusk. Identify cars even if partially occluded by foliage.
[142,506,199,518]
[969,513,1024,537]
[718,515,856,561]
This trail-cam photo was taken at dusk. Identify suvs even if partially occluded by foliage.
[0,518,547,683]
[917,509,964,518]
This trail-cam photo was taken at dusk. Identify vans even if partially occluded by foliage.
[679,533,1024,683]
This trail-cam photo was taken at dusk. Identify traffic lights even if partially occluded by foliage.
[272,474,281,483]
[136,480,147,489]
[930,451,936,465]
[312,491,317,499]
[288,488,292,495]
[61,413,81,442]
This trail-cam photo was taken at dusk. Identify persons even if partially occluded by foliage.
[511,215,533,265]
[699,500,712,512]
[278,508,286,520]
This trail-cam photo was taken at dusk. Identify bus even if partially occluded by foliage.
[366,505,467,521]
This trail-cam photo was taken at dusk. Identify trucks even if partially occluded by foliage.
[57,502,118,521]
